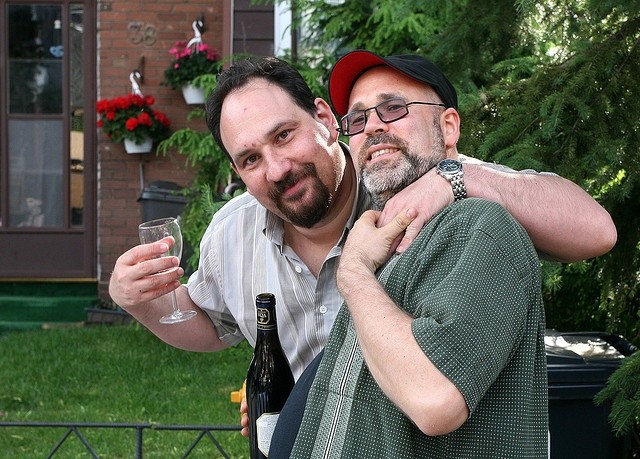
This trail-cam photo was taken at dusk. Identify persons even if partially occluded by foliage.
[241,50,550,459]
[109,56,617,384]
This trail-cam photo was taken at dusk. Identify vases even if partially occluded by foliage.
[183,85,207,105]
[124,138,154,154]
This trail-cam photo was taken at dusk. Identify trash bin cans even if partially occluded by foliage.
[544,330,640,455]
[138,180,192,224]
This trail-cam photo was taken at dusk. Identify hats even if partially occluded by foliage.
[328,49,457,118]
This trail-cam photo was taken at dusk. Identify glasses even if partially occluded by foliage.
[335,97,448,137]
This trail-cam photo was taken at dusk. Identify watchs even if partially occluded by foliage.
[436,159,467,202]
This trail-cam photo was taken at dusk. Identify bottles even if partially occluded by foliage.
[245,293,296,458]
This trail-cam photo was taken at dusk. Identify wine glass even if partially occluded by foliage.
[138,216,198,326]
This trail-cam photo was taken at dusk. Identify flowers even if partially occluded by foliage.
[96,95,170,141]
[160,39,223,91]
